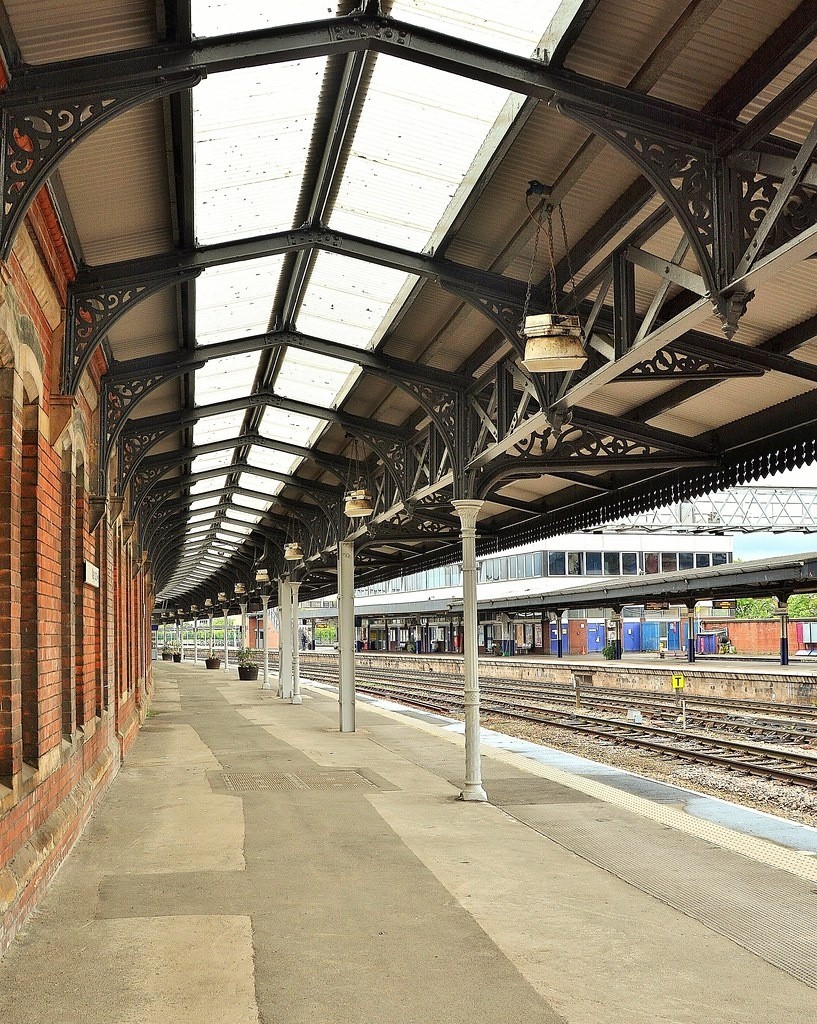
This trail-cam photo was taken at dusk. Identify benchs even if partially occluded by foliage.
[516,643,532,654]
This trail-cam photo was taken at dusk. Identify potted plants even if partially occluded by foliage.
[171,640,181,662]
[205,649,221,669]
[161,647,172,660]
[235,647,259,680]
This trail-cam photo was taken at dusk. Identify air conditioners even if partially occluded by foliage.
[606,619,616,627]
[607,631,619,640]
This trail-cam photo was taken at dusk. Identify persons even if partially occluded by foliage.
[301,633,306,650]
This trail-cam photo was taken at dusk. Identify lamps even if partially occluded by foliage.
[204,599,213,606]
[190,605,198,611]
[177,609,184,614]
[161,613,167,618]
[217,593,227,601]
[169,612,175,617]
[283,513,304,560]
[343,437,374,517]
[233,583,246,593]
[518,192,587,373]
[255,569,269,582]
